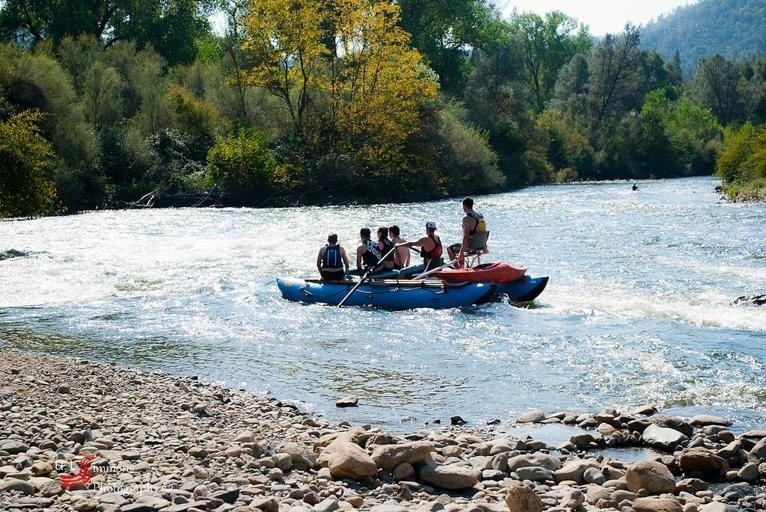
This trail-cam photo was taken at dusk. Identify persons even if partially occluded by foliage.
[315,234,349,280]
[388,224,410,270]
[375,227,394,270]
[446,196,486,268]
[344,227,382,275]
[393,221,442,279]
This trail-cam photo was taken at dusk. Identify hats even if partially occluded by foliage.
[426,222,437,230]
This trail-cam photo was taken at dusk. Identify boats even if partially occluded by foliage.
[274,262,550,314]
[432,260,527,283]
